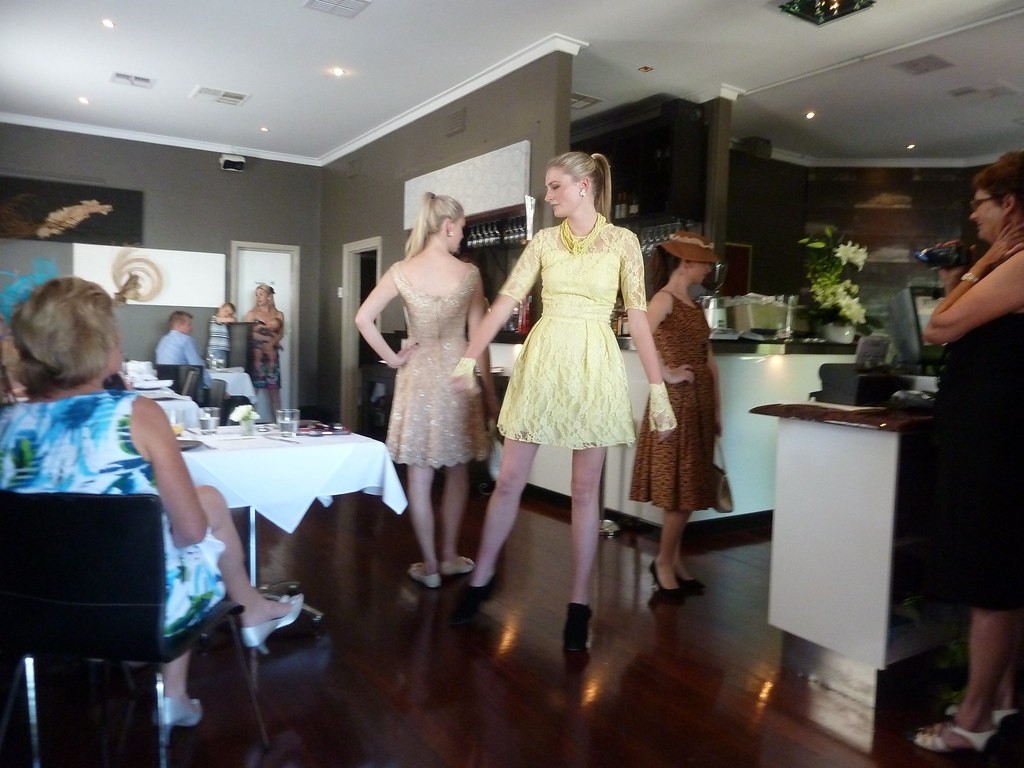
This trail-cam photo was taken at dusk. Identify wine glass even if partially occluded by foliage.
[466,215,527,248]
[640,222,695,257]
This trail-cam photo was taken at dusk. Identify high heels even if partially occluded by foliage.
[650,560,681,599]
[910,720,997,758]
[945,699,1021,730]
[676,566,704,591]
[164,699,202,745]
[563,601,592,655]
[240,592,306,655]
[446,572,496,625]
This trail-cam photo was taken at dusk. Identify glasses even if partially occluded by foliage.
[970,195,997,212]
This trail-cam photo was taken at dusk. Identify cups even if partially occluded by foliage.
[166,409,185,436]
[276,409,300,439]
[198,407,221,436]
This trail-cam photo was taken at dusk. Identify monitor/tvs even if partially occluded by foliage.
[888,284,950,365]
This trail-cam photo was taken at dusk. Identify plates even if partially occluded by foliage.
[177,440,203,451]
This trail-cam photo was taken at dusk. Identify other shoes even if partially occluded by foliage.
[407,561,441,588]
[442,556,475,574]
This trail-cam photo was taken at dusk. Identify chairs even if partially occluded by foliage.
[0,489,273,768]
[219,395,254,426]
[154,362,201,401]
[180,370,199,401]
[206,379,226,407]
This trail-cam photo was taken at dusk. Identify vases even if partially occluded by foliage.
[821,320,856,344]
[239,420,255,435]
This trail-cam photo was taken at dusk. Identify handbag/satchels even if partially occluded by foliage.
[711,464,734,514]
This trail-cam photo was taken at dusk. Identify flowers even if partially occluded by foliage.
[799,225,883,336]
[229,404,262,422]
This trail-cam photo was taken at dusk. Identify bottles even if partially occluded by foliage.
[614,191,639,220]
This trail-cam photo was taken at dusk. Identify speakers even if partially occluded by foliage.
[221,155,246,171]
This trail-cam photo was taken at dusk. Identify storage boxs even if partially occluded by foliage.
[726,303,788,332]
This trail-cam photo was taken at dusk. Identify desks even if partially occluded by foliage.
[203,368,256,407]
[117,370,202,430]
[174,417,409,658]
[358,363,398,437]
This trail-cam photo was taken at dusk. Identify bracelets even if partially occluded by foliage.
[961,273,979,283]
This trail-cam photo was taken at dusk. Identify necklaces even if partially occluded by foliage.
[561,213,606,256]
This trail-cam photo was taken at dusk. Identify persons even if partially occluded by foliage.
[908,151,1024,753]
[1,277,304,742]
[208,302,237,366]
[156,311,249,425]
[245,285,284,417]
[450,152,677,651]
[631,231,723,602]
[355,192,498,587]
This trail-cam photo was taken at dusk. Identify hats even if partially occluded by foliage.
[660,232,721,263]
